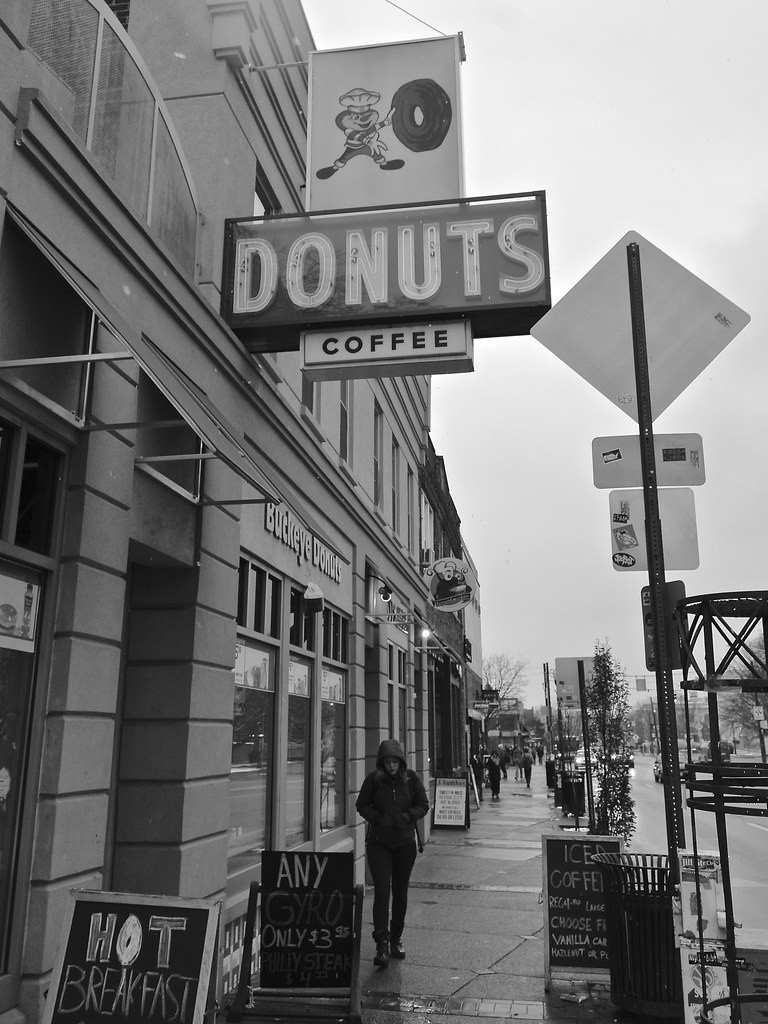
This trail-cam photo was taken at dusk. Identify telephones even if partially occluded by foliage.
[541,835,624,973]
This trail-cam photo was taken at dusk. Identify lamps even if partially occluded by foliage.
[365,572,393,602]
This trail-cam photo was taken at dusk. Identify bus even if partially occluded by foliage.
[705,740,735,763]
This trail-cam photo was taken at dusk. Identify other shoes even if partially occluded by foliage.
[492,797,499,800]
[527,784,530,788]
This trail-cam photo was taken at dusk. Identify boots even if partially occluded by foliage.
[372,921,408,968]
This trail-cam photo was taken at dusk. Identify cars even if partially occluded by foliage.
[574,749,599,770]
[653,752,688,784]
[592,746,601,755]
[595,747,611,763]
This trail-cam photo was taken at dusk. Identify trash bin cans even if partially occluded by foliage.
[545,761,555,786]
[590,850,717,1019]
[562,770,587,817]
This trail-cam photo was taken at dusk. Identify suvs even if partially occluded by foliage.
[610,747,634,770]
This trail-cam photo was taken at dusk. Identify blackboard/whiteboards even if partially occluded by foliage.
[40,888,221,1024]
[433,777,468,826]
[258,849,355,991]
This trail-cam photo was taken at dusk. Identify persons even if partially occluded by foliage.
[497,733,550,788]
[354,738,430,968]
[251,722,265,771]
[485,750,507,800]
[470,750,484,802]
